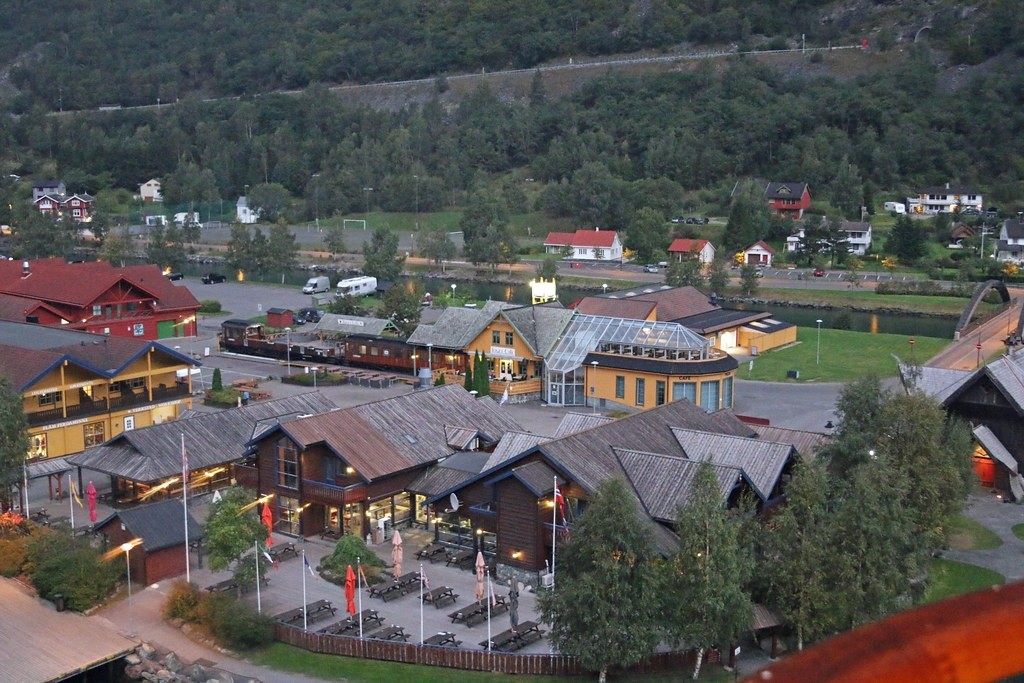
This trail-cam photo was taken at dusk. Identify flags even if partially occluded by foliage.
[359,567,369,590]
[304,556,314,575]
[555,484,568,526]
[257,544,279,570]
[422,569,430,587]
[500,386,509,406]
[183,447,190,480]
[70,481,83,508]
[490,582,497,608]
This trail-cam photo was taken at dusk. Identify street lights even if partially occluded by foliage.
[312,173,321,225]
[602,283,608,294]
[243,184,250,197]
[451,283,457,305]
[413,175,419,232]
[362,187,373,232]
[816,319,822,365]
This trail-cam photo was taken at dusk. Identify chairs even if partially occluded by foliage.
[519,375,524,382]
[524,374,528,380]
[511,373,516,382]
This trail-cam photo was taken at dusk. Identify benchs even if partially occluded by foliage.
[448,602,510,626]
[499,640,522,652]
[343,627,360,636]
[394,634,411,641]
[407,578,429,589]
[449,640,463,647]
[366,627,390,639]
[437,593,459,605]
[444,555,454,562]
[523,630,546,641]
[384,587,407,598]
[290,616,312,626]
[479,628,512,646]
[460,558,473,566]
[431,550,451,559]
[361,616,386,629]
[205,578,271,593]
[312,608,338,618]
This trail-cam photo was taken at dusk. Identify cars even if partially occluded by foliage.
[165,272,184,282]
[200,272,227,285]
[292,313,307,325]
[183,253,341,274]
[813,269,825,277]
[671,216,684,224]
[753,267,763,278]
[298,307,321,323]
[686,216,709,225]
[643,263,659,274]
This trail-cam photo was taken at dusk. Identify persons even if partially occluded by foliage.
[366,532,372,545]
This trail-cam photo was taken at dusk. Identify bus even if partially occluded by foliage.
[335,275,378,299]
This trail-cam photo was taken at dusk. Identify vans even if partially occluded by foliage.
[302,276,330,295]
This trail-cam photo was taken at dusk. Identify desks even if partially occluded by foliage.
[370,571,427,603]
[262,541,299,563]
[97,491,122,505]
[29,505,51,523]
[420,586,456,609]
[367,625,458,648]
[447,602,488,628]
[320,526,343,541]
[476,594,510,618]
[346,608,382,634]
[322,618,359,635]
[41,515,72,530]
[112,495,141,511]
[417,544,473,572]
[302,599,335,624]
[602,344,700,362]
[205,579,245,598]
[70,525,93,538]
[483,621,542,653]
[274,607,310,628]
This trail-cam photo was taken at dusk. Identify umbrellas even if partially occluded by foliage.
[344,565,356,628]
[87,481,97,527]
[476,551,485,605]
[262,504,272,546]
[213,490,223,515]
[509,578,519,630]
[391,530,402,580]
